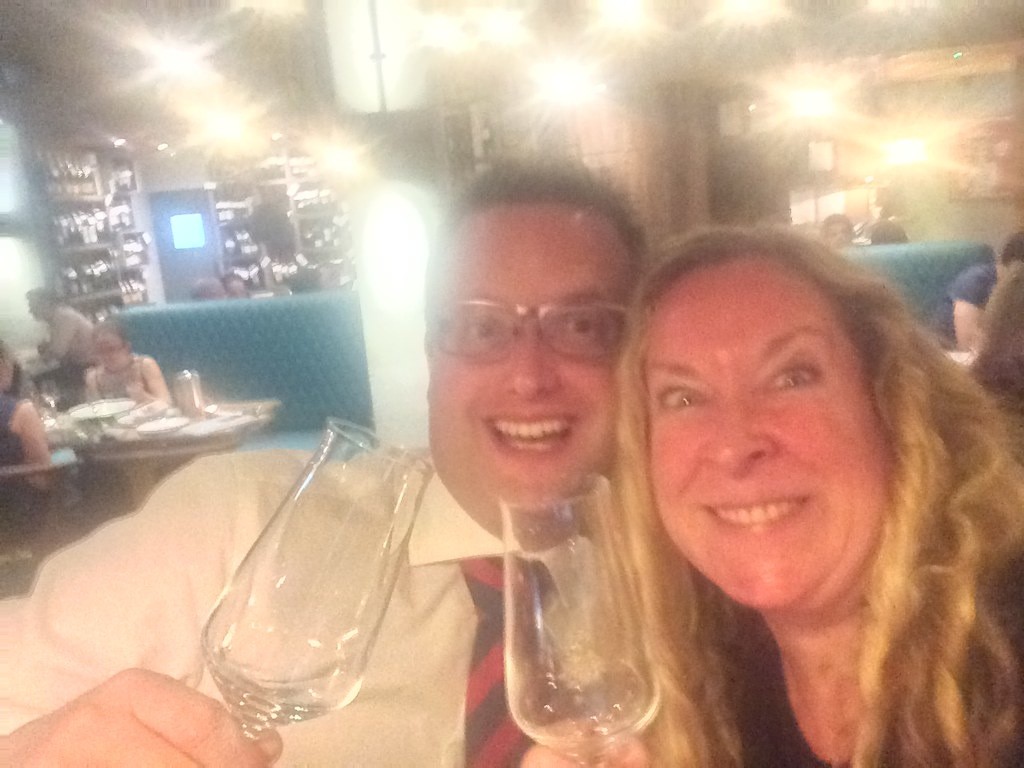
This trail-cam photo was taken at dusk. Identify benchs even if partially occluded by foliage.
[116,289,378,463]
[830,242,998,316]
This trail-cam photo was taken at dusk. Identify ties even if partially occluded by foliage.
[457,555,557,768]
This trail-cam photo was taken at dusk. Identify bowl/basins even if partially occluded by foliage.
[117,415,140,429]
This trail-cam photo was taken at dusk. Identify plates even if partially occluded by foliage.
[67,398,136,419]
[137,416,190,433]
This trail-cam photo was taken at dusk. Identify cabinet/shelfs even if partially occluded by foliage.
[21,136,124,315]
[93,143,152,306]
[204,150,354,295]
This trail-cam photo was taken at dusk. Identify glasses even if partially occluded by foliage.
[427,293,628,363]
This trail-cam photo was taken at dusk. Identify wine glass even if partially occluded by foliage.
[497,472,661,766]
[200,418,435,742]
[40,380,62,419]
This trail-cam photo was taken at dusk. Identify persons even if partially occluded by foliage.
[86,319,173,406]
[26,289,107,502]
[0,341,51,547]
[821,215,854,248]
[0,163,649,768]
[931,232,1024,409]
[521,228,1024,768]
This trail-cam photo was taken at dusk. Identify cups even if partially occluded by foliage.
[173,371,203,418]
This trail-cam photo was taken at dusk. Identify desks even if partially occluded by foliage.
[46,398,283,519]
